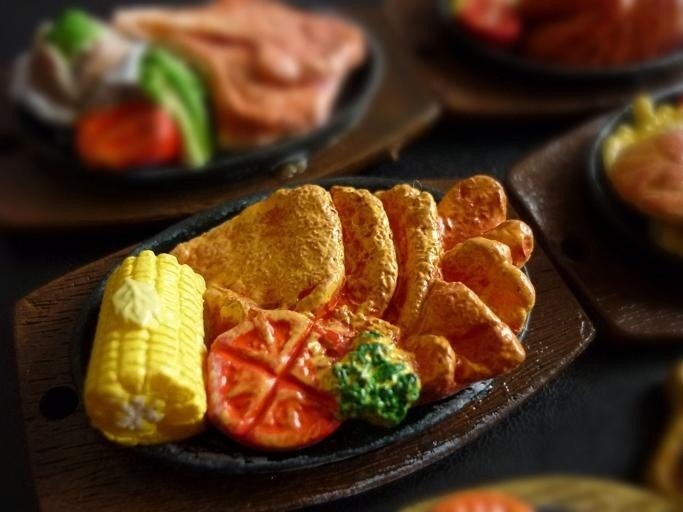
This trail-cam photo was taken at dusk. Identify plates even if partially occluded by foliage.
[432,2,682,91]
[579,82,682,276]
[20,8,386,185]
[67,173,534,466]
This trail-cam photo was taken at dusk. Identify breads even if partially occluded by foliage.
[116,0,363,146]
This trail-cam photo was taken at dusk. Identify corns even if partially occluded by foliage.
[85,250,206,445]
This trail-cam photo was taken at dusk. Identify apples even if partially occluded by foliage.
[76,100,178,168]
